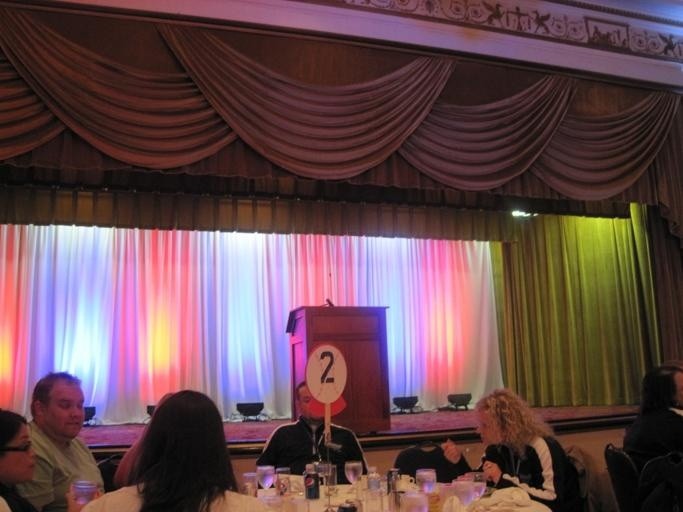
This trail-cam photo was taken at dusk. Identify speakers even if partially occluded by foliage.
[236,402,264,417]
[393,396,417,409]
[448,393,471,405]
[83,406,95,422]
[146,405,156,414]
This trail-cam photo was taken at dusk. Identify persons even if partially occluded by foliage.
[440,387,583,512]
[0,408,40,512]
[621,361,682,485]
[80,390,276,512]
[14,372,105,512]
[254,379,368,490]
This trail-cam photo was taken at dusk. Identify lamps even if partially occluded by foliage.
[391,395,423,416]
[434,392,473,413]
[82,405,96,426]
[142,404,156,424]
[235,401,266,423]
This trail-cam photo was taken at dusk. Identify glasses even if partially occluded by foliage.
[2,441,32,453]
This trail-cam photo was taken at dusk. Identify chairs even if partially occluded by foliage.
[97,452,124,494]
[391,439,472,487]
[565,442,682,512]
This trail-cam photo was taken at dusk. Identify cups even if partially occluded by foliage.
[70,480,97,503]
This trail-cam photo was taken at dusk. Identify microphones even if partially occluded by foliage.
[326,298,333,306]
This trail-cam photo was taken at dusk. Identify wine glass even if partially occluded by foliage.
[240,459,487,512]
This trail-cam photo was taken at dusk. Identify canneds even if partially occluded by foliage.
[305,473,319,499]
[388,469,401,494]
[337,503,357,512]
